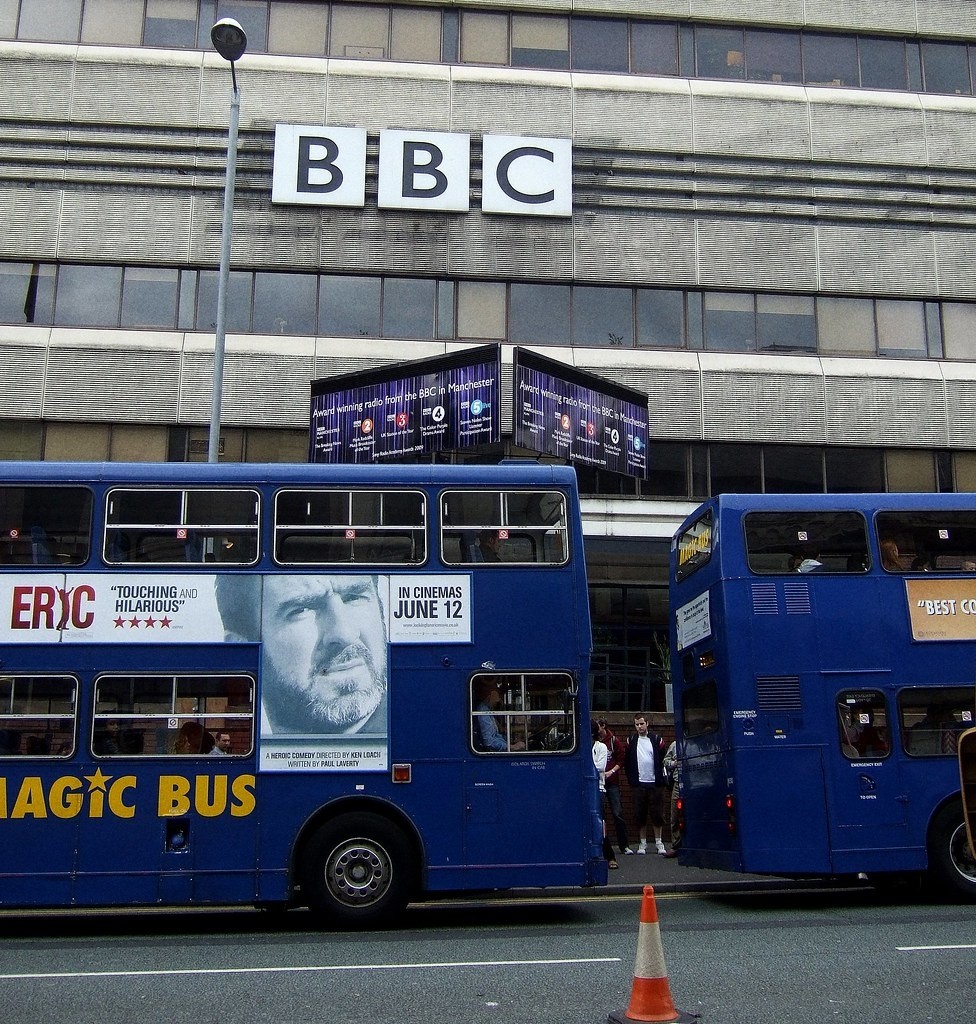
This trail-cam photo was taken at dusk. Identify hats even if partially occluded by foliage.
[182,722,215,754]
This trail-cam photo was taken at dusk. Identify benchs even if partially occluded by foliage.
[279,533,413,563]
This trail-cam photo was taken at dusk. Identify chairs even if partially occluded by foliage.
[106,542,126,562]
[459,532,484,562]
[29,525,63,563]
[185,544,199,561]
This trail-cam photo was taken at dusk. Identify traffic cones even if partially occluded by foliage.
[606,883,698,1024]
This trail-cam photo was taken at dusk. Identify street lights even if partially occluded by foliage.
[205,15,250,463]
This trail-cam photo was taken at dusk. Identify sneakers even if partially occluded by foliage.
[656,843,666,854]
[636,842,648,855]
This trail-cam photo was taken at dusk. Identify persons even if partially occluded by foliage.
[0,537,89,564]
[472,676,526,751]
[57,740,72,756]
[788,533,976,573]
[479,529,502,562]
[105,531,130,562]
[276,502,416,559]
[841,695,976,757]
[215,572,387,735]
[94,709,231,755]
[592,713,680,870]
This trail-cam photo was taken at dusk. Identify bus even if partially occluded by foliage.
[0,460,610,930]
[667,492,976,907]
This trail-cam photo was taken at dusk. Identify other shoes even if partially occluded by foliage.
[608,860,619,870]
[664,848,676,859]
[622,846,635,855]
